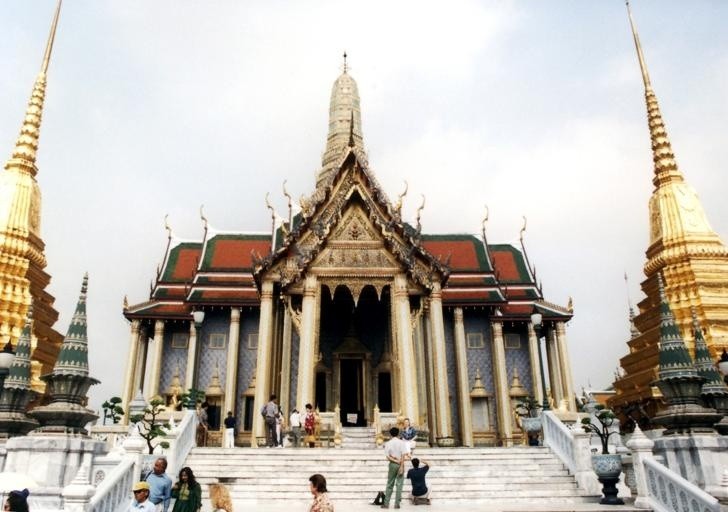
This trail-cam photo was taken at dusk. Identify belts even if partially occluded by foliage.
[292,426,300,428]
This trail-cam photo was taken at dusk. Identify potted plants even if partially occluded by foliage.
[91,397,129,450]
[581,409,627,478]
[130,398,171,475]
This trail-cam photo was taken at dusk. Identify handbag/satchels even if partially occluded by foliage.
[262,407,266,416]
[304,435,316,443]
[374,491,385,503]
[265,417,275,425]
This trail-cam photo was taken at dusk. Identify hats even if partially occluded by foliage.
[131,482,150,491]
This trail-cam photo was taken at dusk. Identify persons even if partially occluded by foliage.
[4,488,30,512]
[196,401,209,447]
[261,394,314,448]
[170,467,202,512]
[224,412,235,448]
[380,427,410,509]
[398,418,417,461]
[309,474,335,512]
[131,458,172,512]
[209,484,233,512]
[406,458,433,505]
[128,481,157,512]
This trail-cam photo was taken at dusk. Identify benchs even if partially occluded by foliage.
[376,421,431,448]
[288,423,334,448]
